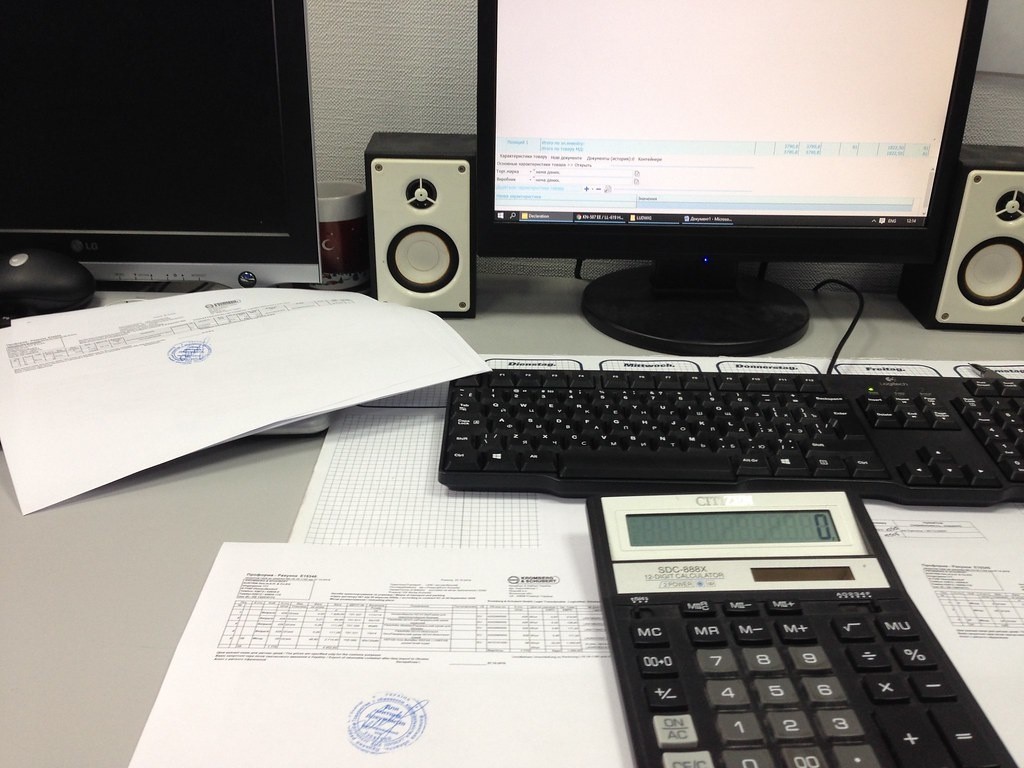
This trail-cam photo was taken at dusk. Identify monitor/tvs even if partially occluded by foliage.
[475,0,990,357]
[0,1,325,293]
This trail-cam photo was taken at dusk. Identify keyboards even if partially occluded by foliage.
[438,368,1024,512]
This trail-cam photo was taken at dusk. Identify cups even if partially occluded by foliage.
[305,180,370,289]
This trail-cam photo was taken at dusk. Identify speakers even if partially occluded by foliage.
[365,131,478,318]
[896,143,1024,332]
